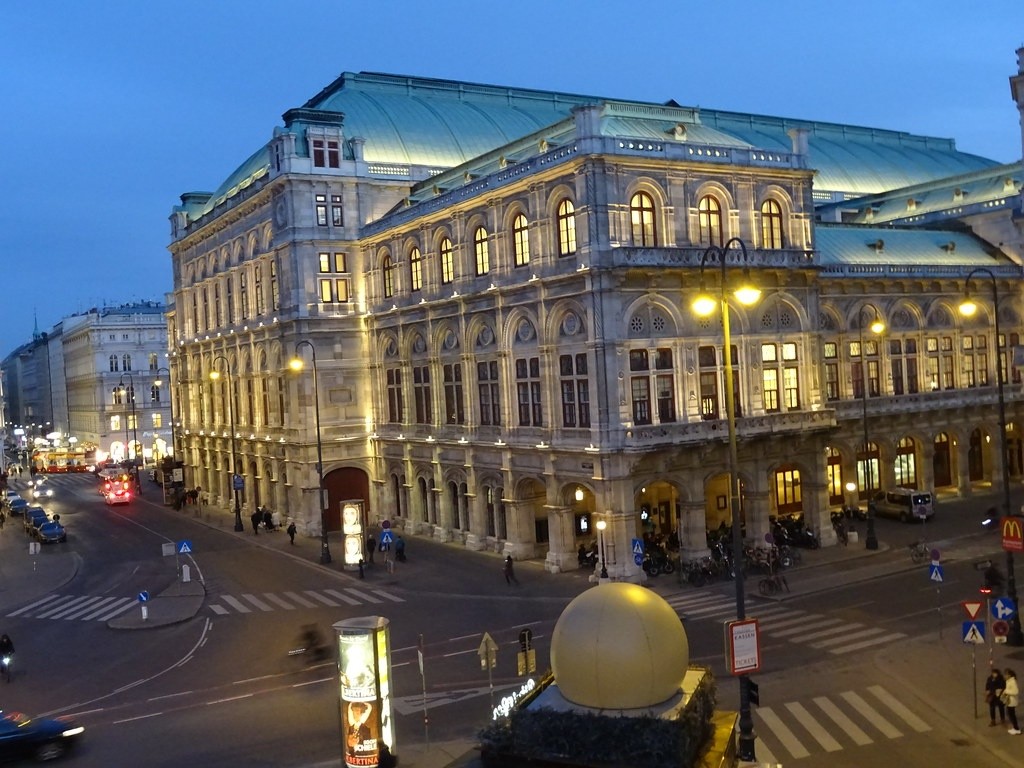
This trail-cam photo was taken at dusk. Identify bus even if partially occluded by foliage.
[32,448,100,474]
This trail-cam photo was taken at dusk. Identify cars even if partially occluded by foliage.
[0,708,80,765]
[28,474,48,489]
[97,459,135,505]
[33,484,52,500]
[25,507,50,533]
[37,521,67,545]
[6,490,30,517]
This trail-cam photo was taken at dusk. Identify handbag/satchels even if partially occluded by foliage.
[996,688,1002,697]
[985,695,992,702]
[1000,692,1008,704]
[263,522,266,526]
[259,521,263,528]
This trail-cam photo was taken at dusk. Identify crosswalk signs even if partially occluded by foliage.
[179,539,192,554]
[381,529,394,545]
[929,564,943,583]
[963,621,986,644]
[631,538,644,555]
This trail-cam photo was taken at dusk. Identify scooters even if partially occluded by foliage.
[578,540,598,567]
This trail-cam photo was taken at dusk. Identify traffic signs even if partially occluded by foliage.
[728,618,760,676]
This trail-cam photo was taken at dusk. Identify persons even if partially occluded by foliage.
[251,505,274,535]
[287,522,297,546]
[986,667,1021,735]
[6,465,39,477]
[95,465,100,478]
[107,455,111,459]
[366,534,375,564]
[731,501,868,547]
[505,556,519,586]
[144,456,148,465]
[167,479,210,510]
[0,498,9,529]
[0,634,15,683]
[394,536,404,557]
[578,544,586,564]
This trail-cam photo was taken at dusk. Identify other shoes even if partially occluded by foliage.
[1009,729,1021,734]
[989,721,996,726]
[1000,720,1005,723]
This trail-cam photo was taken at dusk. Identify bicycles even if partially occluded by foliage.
[830,511,848,547]
[641,513,819,595]
[908,537,930,563]
[0,653,11,684]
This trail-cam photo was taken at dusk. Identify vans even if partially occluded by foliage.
[869,487,936,524]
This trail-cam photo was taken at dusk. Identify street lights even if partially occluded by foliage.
[154,367,176,465]
[858,302,886,535]
[689,237,762,763]
[118,372,143,496]
[957,267,1024,647]
[210,356,244,531]
[290,341,331,566]
